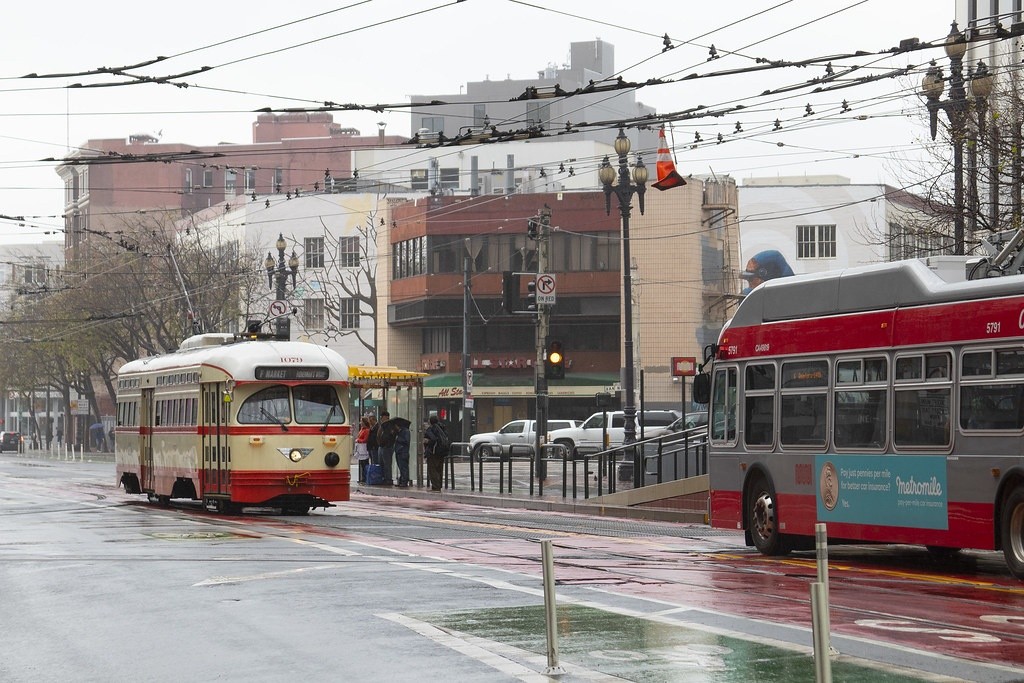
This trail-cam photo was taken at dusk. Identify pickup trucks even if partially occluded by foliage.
[547,410,685,461]
[468,419,582,462]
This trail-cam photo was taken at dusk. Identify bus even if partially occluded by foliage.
[693,256,1024,580]
[114,332,352,516]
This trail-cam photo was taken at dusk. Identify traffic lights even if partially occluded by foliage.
[545,336,566,379]
[528,278,538,318]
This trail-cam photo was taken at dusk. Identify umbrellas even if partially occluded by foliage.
[381,417,412,432]
[89,424,105,430]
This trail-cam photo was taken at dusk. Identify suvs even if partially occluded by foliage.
[0,431,23,453]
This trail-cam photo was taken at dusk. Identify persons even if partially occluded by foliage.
[49,427,63,448]
[423,416,447,493]
[366,416,380,465]
[391,423,410,488]
[96,427,115,452]
[377,411,398,485]
[354,417,370,483]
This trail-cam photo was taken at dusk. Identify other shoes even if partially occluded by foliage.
[426,489,440,492]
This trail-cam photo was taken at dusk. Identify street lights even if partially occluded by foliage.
[922,20,992,258]
[599,128,648,480]
[265,233,299,339]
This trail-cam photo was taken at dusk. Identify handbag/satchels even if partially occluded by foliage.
[353,442,369,460]
[363,465,383,484]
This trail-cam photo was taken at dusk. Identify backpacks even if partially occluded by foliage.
[431,424,453,457]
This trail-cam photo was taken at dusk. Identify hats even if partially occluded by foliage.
[380,411,389,416]
